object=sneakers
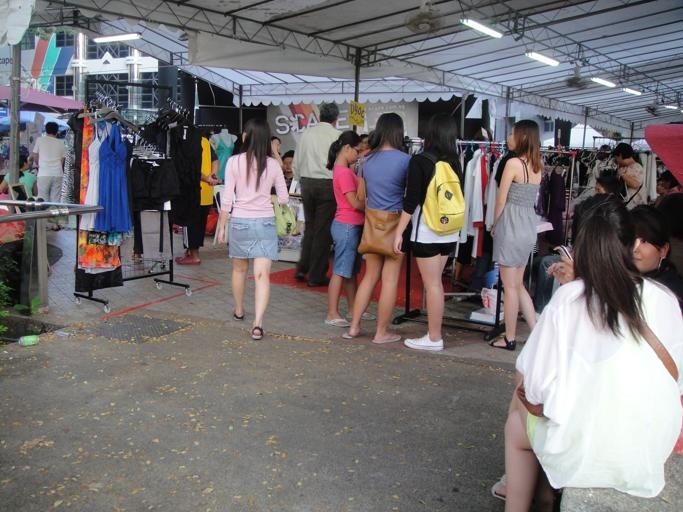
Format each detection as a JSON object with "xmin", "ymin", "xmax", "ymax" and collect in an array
[{"xmin": 402, "ymin": 331, "xmax": 445, "ymax": 352}]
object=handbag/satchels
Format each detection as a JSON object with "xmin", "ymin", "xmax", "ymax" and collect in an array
[
  {"xmin": 357, "ymin": 197, "xmax": 403, "ymax": 261},
  {"xmin": 270, "ymin": 194, "xmax": 297, "ymax": 239},
  {"xmin": 515, "ymin": 377, "xmax": 544, "ymax": 416}
]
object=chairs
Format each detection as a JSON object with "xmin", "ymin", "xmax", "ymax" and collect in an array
[{"xmin": 210, "ymin": 183, "xmax": 236, "ymax": 246}]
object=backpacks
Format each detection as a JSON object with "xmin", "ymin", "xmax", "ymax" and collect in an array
[{"xmin": 417, "ymin": 150, "xmax": 466, "ymax": 236}]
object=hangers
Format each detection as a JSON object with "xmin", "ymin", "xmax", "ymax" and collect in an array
[
  {"xmin": 452, "ymin": 138, "xmax": 600, "ymax": 167},
  {"xmin": 75, "ymin": 91, "xmax": 225, "ymax": 164}
]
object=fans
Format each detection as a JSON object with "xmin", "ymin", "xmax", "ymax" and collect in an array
[{"xmin": 403, "ymin": 0, "xmax": 445, "ymax": 36}]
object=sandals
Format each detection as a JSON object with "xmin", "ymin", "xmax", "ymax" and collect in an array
[
  {"xmin": 491, "ymin": 478, "xmax": 508, "ymax": 502},
  {"xmin": 250, "ymin": 326, "xmax": 265, "ymax": 339},
  {"xmin": 488, "ymin": 335, "xmax": 516, "ymax": 350},
  {"xmin": 233, "ymin": 308, "xmax": 246, "ymax": 321},
  {"xmin": 45, "ymin": 224, "xmax": 61, "ymax": 231}
]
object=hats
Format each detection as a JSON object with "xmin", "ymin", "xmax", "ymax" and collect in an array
[{"xmin": 626, "ymin": 204, "xmax": 671, "ymax": 249}]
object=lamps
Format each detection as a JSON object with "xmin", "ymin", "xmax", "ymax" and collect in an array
[
  {"xmin": 589, "ymin": 69, "xmax": 617, "ymax": 89},
  {"xmin": 621, "ymin": 80, "xmax": 641, "ymax": 97},
  {"xmin": 458, "ymin": 8, "xmax": 504, "ymax": 40},
  {"xmin": 91, "ymin": 20, "xmax": 148, "ymax": 44},
  {"xmin": 523, "ymin": 40, "xmax": 560, "ymax": 68}
]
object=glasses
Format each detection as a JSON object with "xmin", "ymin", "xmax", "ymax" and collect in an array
[{"xmin": 353, "ymin": 147, "xmax": 360, "ymax": 155}]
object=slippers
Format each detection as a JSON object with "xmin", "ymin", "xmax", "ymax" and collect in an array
[
  {"xmin": 346, "ymin": 312, "xmax": 376, "ymax": 320},
  {"xmin": 174, "ymin": 255, "xmax": 201, "ymax": 264},
  {"xmin": 342, "ymin": 328, "xmax": 368, "ymax": 339},
  {"xmin": 372, "ymin": 333, "xmax": 401, "ymax": 344},
  {"xmin": 324, "ymin": 316, "xmax": 351, "ymax": 327}
]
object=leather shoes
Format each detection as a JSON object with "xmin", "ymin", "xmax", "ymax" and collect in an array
[
  {"xmin": 307, "ymin": 277, "xmax": 330, "ymax": 287},
  {"xmin": 293, "ymin": 271, "xmax": 305, "ymax": 281}
]
